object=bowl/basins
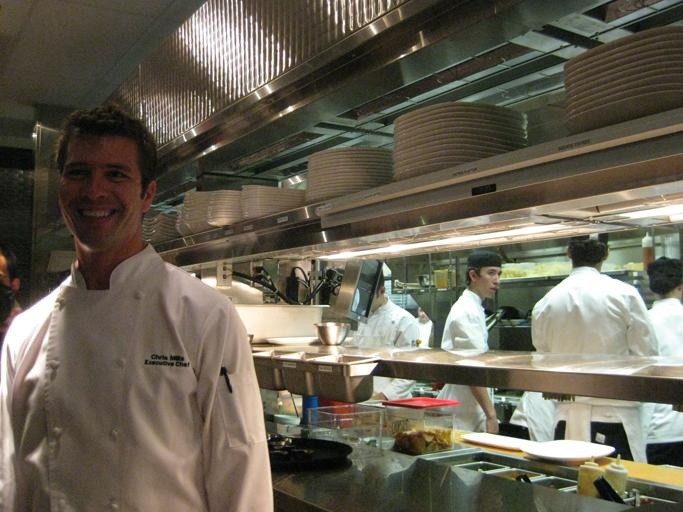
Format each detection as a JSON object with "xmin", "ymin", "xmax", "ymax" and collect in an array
[
  {"xmin": 363, "ymin": 437, "xmax": 395, "ymax": 450},
  {"xmin": 315, "ymin": 321, "xmax": 351, "ymax": 346}
]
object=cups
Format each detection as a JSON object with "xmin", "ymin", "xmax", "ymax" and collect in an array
[{"xmin": 272, "ymin": 413, "xmax": 300, "ymax": 426}]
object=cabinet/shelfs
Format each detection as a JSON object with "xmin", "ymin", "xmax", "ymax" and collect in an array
[
  {"xmin": 32, "ymin": 19, "xmax": 682, "ymax": 510},
  {"xmin": 398, "ymin": 224, "xmax": 682, "ymax": 467}
]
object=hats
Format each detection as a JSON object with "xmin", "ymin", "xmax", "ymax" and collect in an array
[{"xmin": 468, "ymin": 250, "xmax": 503, "ymax": 268}]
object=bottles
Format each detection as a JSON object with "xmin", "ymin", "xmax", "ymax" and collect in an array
[
  {"xmin": 641, "ymin": 231, "xmax": 652, "ymax": 271},
  {"xmin": 605, "ymin": 452, "xmax": 626, "ymax": 497},
  {"xmin": 577, "ymin": 455, "xmax": 602, "ymax": 499}
]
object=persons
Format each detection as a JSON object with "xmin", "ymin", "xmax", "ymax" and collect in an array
[
  {"xmin": 436, "ymin": 250, "xmax": 501, "ymax": 434},
  {"xmin": 350, "ymin": 268, "xmax": 419, "ymax": 403},
  {"xmin": 638, "ymin": 256, "xmax": 683, "ymax": 468},
  {"xmin": 416, "ymin": 307, "xmax": 433, "ymax": 351},
  {"xmin": 0, "ymin": 104, "xmax": 277, "ymax": 511},
  {"xmin": 524, "ymin": 233, "xmax": 659, "ymax": 464},
  {"xmin": 0, "ymin": 244, "xmax": 25, "ymax": 335}
]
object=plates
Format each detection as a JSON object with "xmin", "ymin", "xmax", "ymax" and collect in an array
[
  {"xmin": 389, "ymin": 101, "xmax": 527, "ymax": 180},
  {"xmin": 140, "ymin": 211, "xmax": 176, "ymax": 245},
  {"xmin": 520, "ymin": 438, "xmax": 615, "ymax": 464},
  {"xmin": 463, "ymin": 432, "xmax": 537, "ymax": 451},
  {"xmin": 265, "ymin": 337, "xmax": 318, "ymax": 346},
  {"xmin": 560, "ymin": 24, "xmax": 683, "ymax": 134},
  {"xmin": 302, "ymin": 145, "xmax": 389, "ymax": 205},
  {"xmin": 175, "ymin": 184, "xmax": 304, "ymax": 236}
]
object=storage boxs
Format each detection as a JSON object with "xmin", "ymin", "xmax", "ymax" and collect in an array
[
  {"xmin": 308, "ymin": 400, "xmax": 384, "ymax": 446},
  {"xmin": 383, "ymin": 396, "xmax": 459, "ymax": 455}
]
